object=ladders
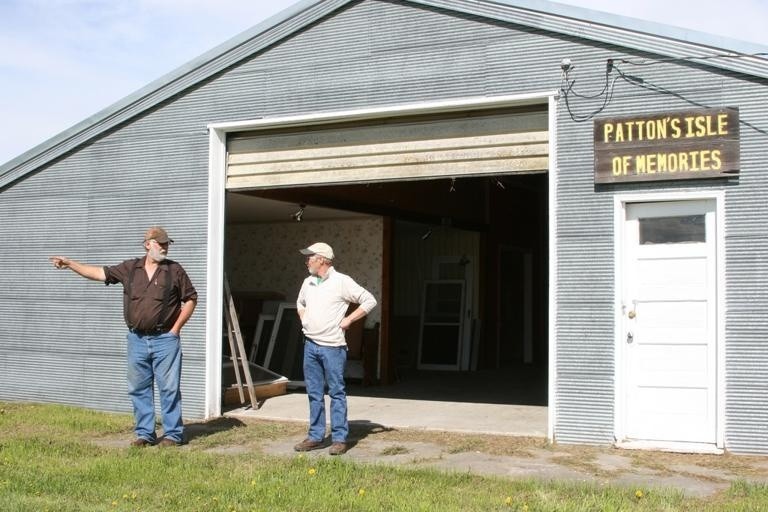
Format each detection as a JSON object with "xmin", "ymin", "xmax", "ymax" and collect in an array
[{"xmin": 224, "ymin": 273, "xmax": 258, "ymax": 410}]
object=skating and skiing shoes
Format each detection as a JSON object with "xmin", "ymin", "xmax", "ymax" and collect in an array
[{"xmin": 130, "ymin": 436, "xmax": 154, "ymax": 448}]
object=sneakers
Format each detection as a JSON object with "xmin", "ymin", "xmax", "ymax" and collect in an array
[
  {"xmin": 329, "ymin": 438, "xmax": 352, "ymax": 455},
  {"xmin": 293, "ymin": 435, "xmax": 327, "ymax": 451},
  {"xmin": 157, "ymin": 435, "xmax": 184, "ymax": 448}
]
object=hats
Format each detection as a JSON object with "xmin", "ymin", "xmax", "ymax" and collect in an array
[
  {"xmin": 296, "ymin": 241, "xmax": 338, "ymax": 261},
  {"xmin": 142, "ymin": 225, "xmax": 175, "ymax": 244}
]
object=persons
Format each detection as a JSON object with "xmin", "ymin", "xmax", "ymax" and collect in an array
[
  {"xmin": 48, "ymin": 225, "xmax": 198, "ymax": 447},
  {"xmin": 295, "ymin": 241, "xmax": 377, "ymax": 454}
]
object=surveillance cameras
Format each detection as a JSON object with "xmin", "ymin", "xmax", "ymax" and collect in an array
[{"xmin": 561, "ymin": 58, "xmax": 570, "ymax": 70}]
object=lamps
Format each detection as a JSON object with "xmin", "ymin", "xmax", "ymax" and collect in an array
[{"xmin": 288, "ymin": 201, "xmax": 306, "ymax": 222}]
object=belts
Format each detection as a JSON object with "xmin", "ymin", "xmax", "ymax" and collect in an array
[
  {"xmin": 303, "ymin": 334, "xmax": 313, "ymax": 342},
  {"xmin": 129, "ymin": 327, "xmax": 170, "ymax": 338}
]
